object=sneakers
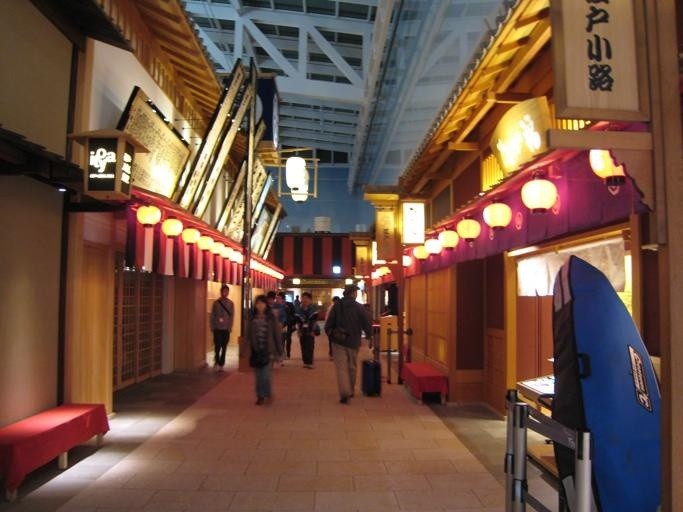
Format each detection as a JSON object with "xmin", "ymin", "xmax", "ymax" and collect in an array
[
  {"xmin": 304, "ymin": 364, "xmax": 313, "ymax": 369},
  {"xmin": 341, "ymin": 398, "xmax": 348, "ymax": 403},
  {"xmin": 255, "ymin": 396, "xmax": 264, "ymax": 406}
]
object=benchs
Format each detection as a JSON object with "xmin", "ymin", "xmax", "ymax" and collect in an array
[
  {"xmin": 0, "ymin": 402, "xmax": 110, "ymax": 501},
  {"xmin": 400, "ymin": 361, "xmax": 448, "ymax": 405}
]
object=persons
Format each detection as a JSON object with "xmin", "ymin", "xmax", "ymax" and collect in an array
[
  {"xmin": 265, "ymin": 291, "xmax": 286, "ymax": 367},
  {"xmin": 323, "ymin": 286, "xmax": 374, "ymax": 402},
  {"xmin": 211, "ymin": 286, "xmax": 234, "ymax": 373},
  {"xmin": 277, "ymin": 293, "xmax": 294, "ymax": 359},
  {"xmin": 295, "ymin": 296, "xmax": 300, "ymax": 312},
  {"xmin": 295, "ymin": 292, "xmax": 319, "ymax": 368},
  {"xmin": 243, "ymin": 294, "xmax": 281, "ymax": 405},
  {"xmin": 326, "ymin": 292, "xmax": 340, "ymax": 361}
]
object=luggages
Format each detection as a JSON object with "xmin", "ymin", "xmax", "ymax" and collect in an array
[{"xmin": 361, "ymin": 360, "xmax": 381, "ymax": 397}]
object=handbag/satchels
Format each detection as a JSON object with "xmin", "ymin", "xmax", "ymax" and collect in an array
[
  {"xmin": 314, "ymin": 325, "xmax": 320, "ymax": 336},
  {"xmin": 250, "ymin": 350, "xmax": 268, "ymax": 368},
  {"xmin": 332, "ymin": 328, "xmax": 348, "ymax": 346}
]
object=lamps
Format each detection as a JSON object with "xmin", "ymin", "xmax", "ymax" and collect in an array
[
  {"xmin": 372, "ymin": 149, "xmax": 626, "ymax": 277},
  {"xmin": 67, "ymin": 128, "xmax": 151, "ymax": 201},
  {"xmin": 136, "ymin": 205, "xmax": 284, "ymax": 280}
]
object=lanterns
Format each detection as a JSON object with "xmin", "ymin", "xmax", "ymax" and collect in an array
[
  {"xmin": 181, "ymin": 227, "xmax": 200, "ymax": 247},
  {"xmin": 213, "ymin": 242, "xmax": 289, "ymax": 281},
  {"xmin": 161, "ymin": 217, "xmax": 185, "ymax": 239},
  {"xmin": 286, "ymin": 156, "xmax": 309, "ymax": 204},
  {"xmin": 520, "ymin": 180, "xmax": 557, "ymax": 214},
  {"xmin": 482, "ymin": 204, "xmax": 512, "ymax": 231},
  {"xmin": 438, "ymin": 230, "xmax": 457, "ymax": 251},
  {"xmin": 589, "ymin": 148, "xmax": 625, "ymax": 189},
  {"xmin": 136, "ymin": 202, "xmax": 162, "ymax": 227},
  {"xmin": 198, "ymin": 234, "xmax": 215, "ymax": 251},
  {"xmin": 367, "ymin": 237, "xmax": 442, "ymax": 280},
  {"xmin": 455, "ymin": 219, "xmax": 481, "ymax": 242}
]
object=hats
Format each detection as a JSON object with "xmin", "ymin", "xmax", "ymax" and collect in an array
[{"xmin": 344, "ymin": 285, "xmax": 361, "ymax": 293}]
